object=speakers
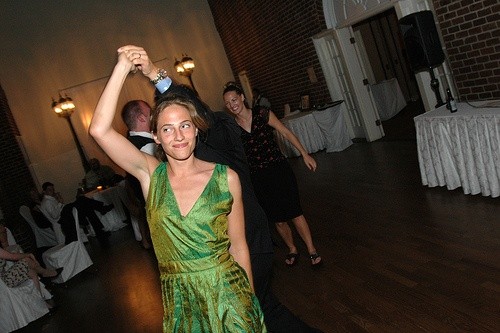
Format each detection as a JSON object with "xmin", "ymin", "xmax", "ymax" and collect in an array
[{"xmin": 398, "ymin": 10, "xmax": 445, "ymax": 74}]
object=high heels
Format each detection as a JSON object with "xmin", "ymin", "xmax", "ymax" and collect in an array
[{"xmin": 44, "ymin": 267, "xmax": 64, "ymax": 282}]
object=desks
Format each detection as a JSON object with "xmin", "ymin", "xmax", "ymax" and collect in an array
[
  {"xmin": 272, "ymin": 110, "xmax": 326, "ymax": 157},
  {"xmin": 413, "ymin": 100, "xmax": 499, "ymax": 199},
  {"xmin": 85, "ymin": 184, "xmax": 128, "ymax": 231}
]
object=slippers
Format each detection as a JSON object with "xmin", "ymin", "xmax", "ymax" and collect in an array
[
  {"xmin": 285, "ymin": 252, "xmax": 300, "ymax": 267},
  {"xmin": 310, "ymin": 252, "xmax": 322, "ymax": 270}
]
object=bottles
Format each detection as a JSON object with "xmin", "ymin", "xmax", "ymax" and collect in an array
[{"xmin": 446, "ymin": 89, "xmax": 457, "ymax": 113}]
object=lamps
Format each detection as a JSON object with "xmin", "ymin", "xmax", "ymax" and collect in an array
[
  {"xmin": 174, "ymin": 52, "xmax": 195, "ymax": 77},
  {"xmin": 51, "ymin": 93, "xmax": 76, "ymax": 117}
]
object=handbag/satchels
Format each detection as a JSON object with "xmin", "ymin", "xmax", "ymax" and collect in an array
[{"xmin": 3, "ymin": 261, "xmax": 30, "ymax": 287}]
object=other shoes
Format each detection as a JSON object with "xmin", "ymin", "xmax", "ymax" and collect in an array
[
  {"xmin": 100, "ymin": 203, "xmax": 115, "ymax": 215},
  {"xmin": 96, "ymin": 230, "xmax": 111, "ymax": 238},
  {"xmin": 141, "ymin": 241, "xmax": 152, "ymax": 251}
]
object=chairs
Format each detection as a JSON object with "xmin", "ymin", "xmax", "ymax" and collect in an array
[
  {"xmin": 36, "ymin": 203, "xmax": 94, "ymax": 243},
  {"xmin": 42, "ymin": 208, "xmax": 94, "ymax": 283},
  {"xmin": 117, "ymin": 179, "xmax": 143, "ymax": 241},
  {"xmin": 18, "ymin": 205, "xmax": 55, "ymax": 246},
  {"xmin": 0, "ymin": 227, "xmax": 53, "ymax": 333}
]
